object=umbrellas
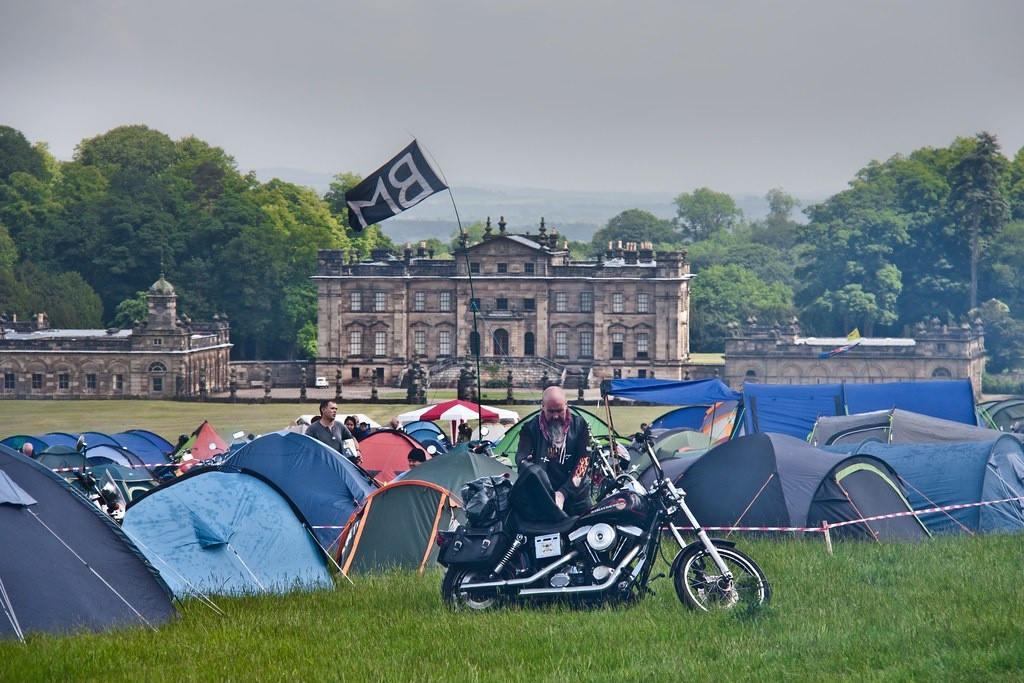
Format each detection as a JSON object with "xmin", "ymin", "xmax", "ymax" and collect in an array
[{"xmin": 398, "ymin": 399, "xmax": 519, "ymax": 445}]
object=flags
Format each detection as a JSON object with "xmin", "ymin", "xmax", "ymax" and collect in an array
[{"xmin": 344, "ymin": 140, "xmax": 448, "ymax": 234}]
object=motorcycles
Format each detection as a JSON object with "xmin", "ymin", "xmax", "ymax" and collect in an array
[{"xmin": 435, "ymin": 422, "xmax": 773, "ymax": 624}]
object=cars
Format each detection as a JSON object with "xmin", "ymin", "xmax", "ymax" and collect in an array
[{"xmin": 315, "ymin": 376, "xmax": 330, "ymax": 389}]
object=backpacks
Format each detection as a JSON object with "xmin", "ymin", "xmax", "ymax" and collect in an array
[{"xmin": 461, "ymin": 471, "xmax": 513, "ymax": 528}]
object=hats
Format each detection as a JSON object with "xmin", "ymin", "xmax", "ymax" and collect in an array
[{"xmin": 407, "ymin": 448, "xmax": 426, "ymax": 461}]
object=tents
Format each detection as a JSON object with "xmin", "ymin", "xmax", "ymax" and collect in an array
[
  {"xmin": 493, "ymin": 404, "xmax": 619, "ymax": 473},
  {"xmin": 600, "ymin": 377, "xmax": 1024, "ymax": 544},
  {"xmin": 0, "ymin": 442, "xmax": 179, "ymax": 643},
  {"xmin": 337, "ymin": 451, "xmax": 520, "ymax": 572},
  {"xmin": 285, "ymin": 413, "xmax": 381, "ymax": 434},
  {"xmin": 403, "ymin": 421, "xmax": 453, "ymax": 456},
  {"xmin": 355, "ymin": 429, "xmax": 434, "ymax": 485},
  {"xmin": 0, "ymin": 421, "xmax": 378, "ymax": 596}
]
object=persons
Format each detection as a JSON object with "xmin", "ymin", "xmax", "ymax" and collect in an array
[
  {"xmin": 170, "ymin": 434, "xmax": 189, "ymax": 456},
  {"xmin": 641, "ymin": 423, "xmax": 652, "ymax": 435},
  {"xmin": 22, "ymin": 442, "xmax": 34, "ymax": 457},
  {"xmin": 407, "ymin": 449, "xmax": 427, "ymax": 469},
  {"xmin": 516, "ymin": 386, "xmax": 594, "ymax": 518},
  {"xmin": 343, "ymin": 415, "xmax": 370, "ymax": 442},
  {"xmin": 390, "ymin": 418, "xmax": 408, "ymax": 434},
  {"xmin": 305, "ymin": 400, "xmax": 363, "ymax": 466}
]
